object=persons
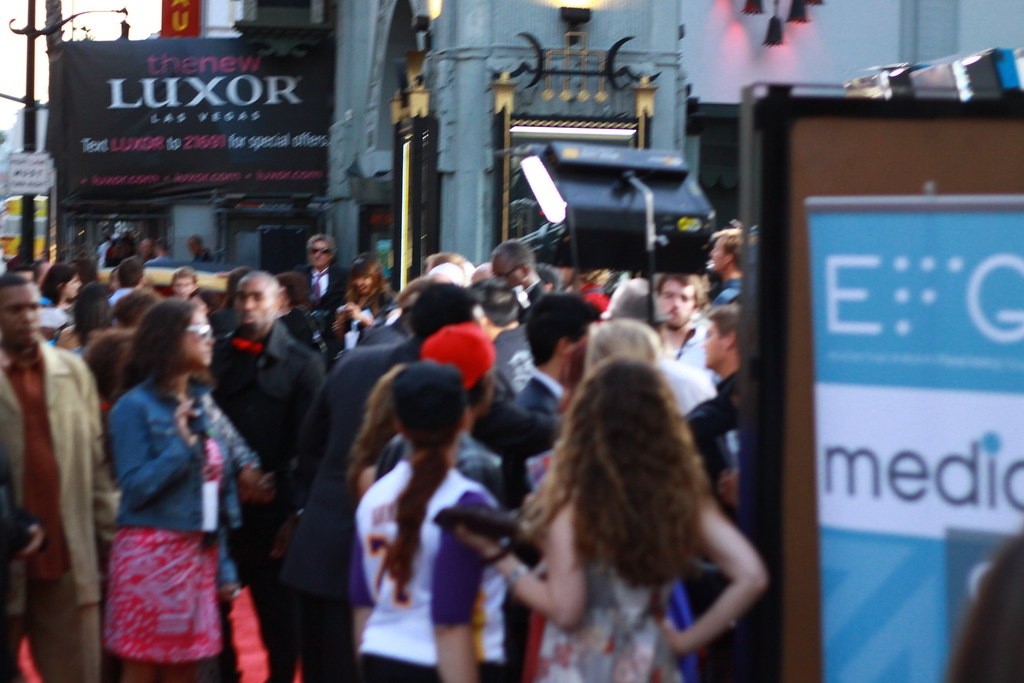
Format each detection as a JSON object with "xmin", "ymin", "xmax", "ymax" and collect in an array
[
  {"xmin": 103, "ymin": 297, "xmax": 277, "ymax": 683},
  {"xmin": 451, "ymin": 354, "xmax": 771, "ymax": 683},
  {"xmin": 0, "ymin": 222, "xmax": 770, "ymax": 683}
]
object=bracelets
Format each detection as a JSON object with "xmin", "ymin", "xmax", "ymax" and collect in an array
[{"xmin": 483, "ymin": 544, "xmax": 510, "ymax": 564}]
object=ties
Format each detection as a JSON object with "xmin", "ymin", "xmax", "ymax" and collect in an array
[{"xmin": 314, "ymin": 270, "xmax": 329, "ymax": 298}]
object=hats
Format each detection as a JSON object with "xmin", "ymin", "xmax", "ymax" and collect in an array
[
  {"xmin": 393, "ymin": 360, "xmax": 474, "ymax": 429},
  {"xmin": 421, "ymin": 323, "xmax": 497, "ymax": 391}
]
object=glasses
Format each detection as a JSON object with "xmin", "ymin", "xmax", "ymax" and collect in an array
[
  {"xmin": 188, "ymin": 324, "xmax": 213, "ymax": 339},
  {"xmin": 311, "ymin": 247, "xmax": 330, "ymax": 253},
  {"xmin": 495, "ymin": 265, "xmax": 520, "ymax": 280}
]
